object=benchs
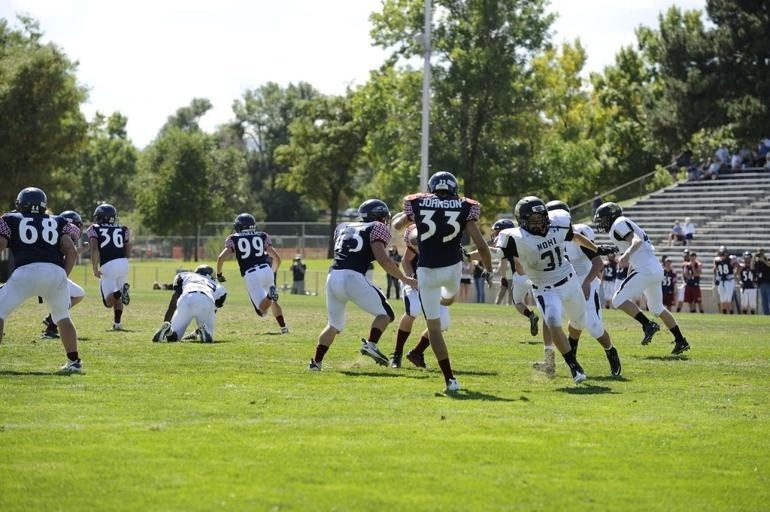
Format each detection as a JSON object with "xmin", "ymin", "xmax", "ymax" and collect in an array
[{"xmin": 591, "ymin": 169, "xmax": 770, "ymax": 288}]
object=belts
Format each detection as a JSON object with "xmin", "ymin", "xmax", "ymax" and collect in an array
[
  {"xmin": 532, "ymin": 273, "xmax": 572, "ymax": 289},
  {"xmin": 248, "ymin": 265, "xmax": 267, "ymax": 273}
]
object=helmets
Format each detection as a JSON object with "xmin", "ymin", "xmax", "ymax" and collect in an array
[
  {"xmin": 196, "ymin": 266, "xmax": 216, "ymax": 280},
  {"xmin": 234, "ymin": 213, "xmax": 256, "ymax": 234},
  {"xmin": 16, "ymin": 187, "xmax": 116, "ymax": 229},
  {"xmin": 357, "ymin": 172, "xmax": 623, "ymax": 237},
  {"xmin": 663, "ymin": 245, "xmax": 765, "ymax": 263}
]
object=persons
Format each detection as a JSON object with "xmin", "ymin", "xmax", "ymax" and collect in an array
[
  {"xmin": 665, "ymin": 134, "xmax": 770, "ymax": 184},
  {"xmin": 385, "ymin": 211, "xmax": 769, "ymax": 379},
  {"xmin": 289, "ymin": 254, "xmax": 306, "ymax": 296},
  {"xmin": 306, "ymin": 197, "xmax": 418, "ymax": 371},
  {"xmin": 0, "ymin": 186, "xmax": 174, "ymax": 377},
  {"xmin": 541, "ymin": 200, "xmax": 622, "ymax": 378},
  {"xmin": 215, "ymin": 212, "xmax": 289, "ymax": 334},
  {"xmin": 390, "ymin": 171, "xmax": 493, "ymax": 392},
  {"xmin": 591, "ymin": 201, "xmax": 691, "ymax": 356},
  {"xmin": 152, "ymin": 265, "xmax": 228, "ymax": 343},
  {"xmin": 495, "ymin": 194, "xmax": 614, "ymax": 384},
  {"xmin": 593, "ymin": 190, "xmax": 603, "ymax": 209}
]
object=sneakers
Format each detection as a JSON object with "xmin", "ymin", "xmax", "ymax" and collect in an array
[{"xmin": 39, "ymin": 284, "xmax": 291, "ymax": 374}]
